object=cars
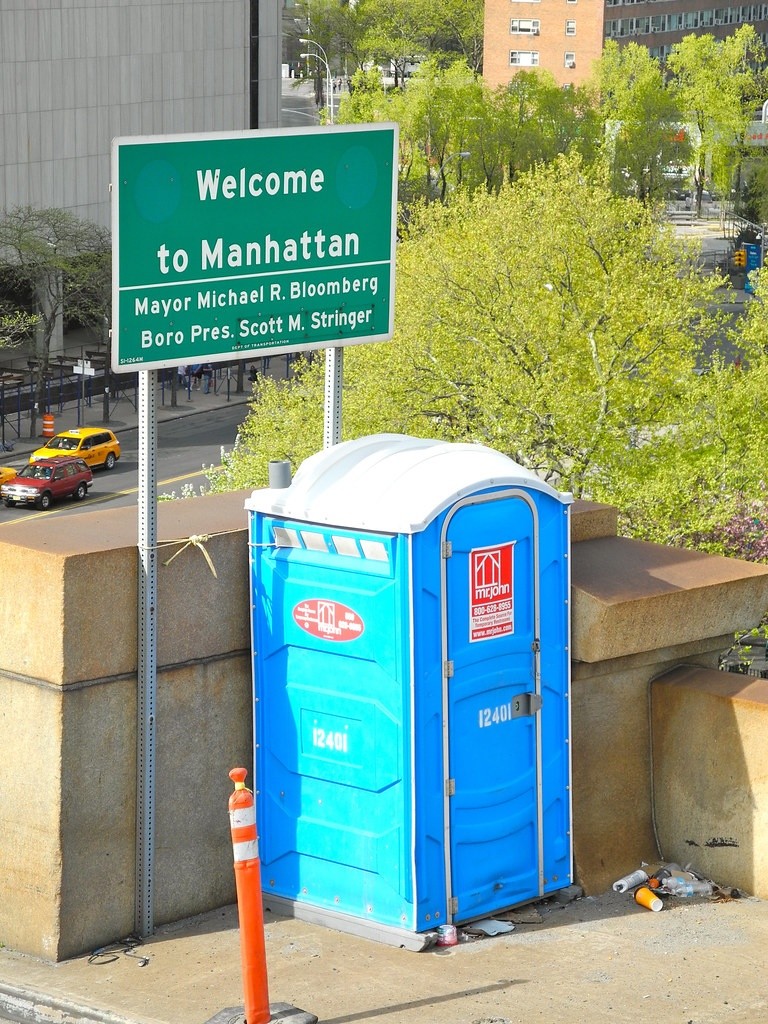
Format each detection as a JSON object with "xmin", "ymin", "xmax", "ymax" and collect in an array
[{"xmin": 0, "ymin": 466, "xmax": 19, "ymax": 498}]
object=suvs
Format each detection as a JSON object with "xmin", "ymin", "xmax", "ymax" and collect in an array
[
  {"xmin": 28, "ymin": 425, "xmax": 121, "ymax": 471},
  {"xmin": 0, "ymin": 455, "xmax": 93, "ymax": 511}
]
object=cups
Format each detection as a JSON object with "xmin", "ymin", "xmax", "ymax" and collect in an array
[
  {"xmin": 437, "ymin": 925, "xmax": 457, "ymax": 947},
  {"xmin": 634, "ymin": 885, "xmax": 663, "ymax": 912}
]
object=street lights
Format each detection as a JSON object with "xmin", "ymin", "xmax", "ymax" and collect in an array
[
  {"xmin": 755, "ymin": 222, "xmax": 766, "ymax": 276},
  {"xmin": 299, "ymin": 38, "xmax": 329, "ymax": 127},
  {"xmin": 300, "ymin": 52, "xmax": 334, "ymax": 126}
]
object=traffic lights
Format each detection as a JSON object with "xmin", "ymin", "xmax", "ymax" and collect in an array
[{"xmin": 734, "ymin": 249, "xmax": 747, "ymax": 266}]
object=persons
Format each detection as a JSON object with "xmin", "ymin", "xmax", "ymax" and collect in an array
[
  {"xmin": 177, "ymin": 363, "xmax": 212, "ymax": 394},
  {"xmin": 247, "ymin": 365, "xmax": 259, "ymax": 383}
]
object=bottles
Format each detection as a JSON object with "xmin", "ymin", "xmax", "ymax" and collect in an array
[
  {"xmin": 648, "ymin": 862, "xmax": 681, "ymax": 889},
  {"xmin": 671, "ymin": 884, "xmax": 713, "ymax": 898},
  {"xmin": 662, "ymin": 877, "xmax": 707, "ymax": 889},
  {"xmin": 613, "ymin": 870, "xmax": 648, "ymax": 893}
]
